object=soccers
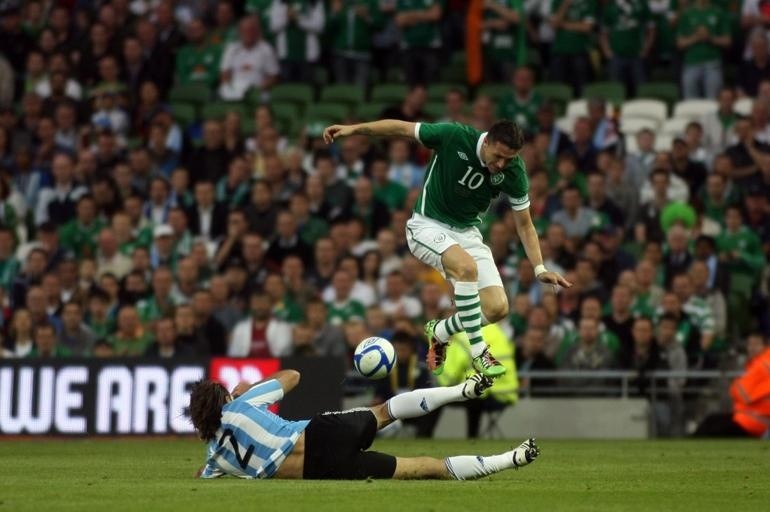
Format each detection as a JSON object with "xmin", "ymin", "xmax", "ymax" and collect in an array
[{"xmin": 354, "ymin": 337, "xmax": 397, "ymax": 379}]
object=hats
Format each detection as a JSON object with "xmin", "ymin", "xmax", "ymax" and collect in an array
[{"xmin": 153, "ymin": 225, "xmax": 174, "ymax": 237}]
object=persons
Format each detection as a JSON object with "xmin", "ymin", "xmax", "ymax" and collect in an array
[
  {"xmin": 0, "ymin": 1, "xmax": 769, "ymax": 438},
  {"xmin": 186, "ymin": 370, "xmax": 540, "ymax": 480}
]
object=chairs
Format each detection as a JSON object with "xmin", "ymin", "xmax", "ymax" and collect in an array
[{"xmin": 162, "ymin": 41, "xmax": 740, "ymax": 174}]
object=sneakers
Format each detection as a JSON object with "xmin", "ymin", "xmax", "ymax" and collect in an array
[
  {"xmin": 462, "ymin": 345, "xmax": 506, "ymax": 399},
  {"xmin": 424, "ymin": 319, "xmax": 447, "ymax": 375},
  {"xmin": 513, "ymin": 438, "xmax": 540, "ymax": 466}
]
